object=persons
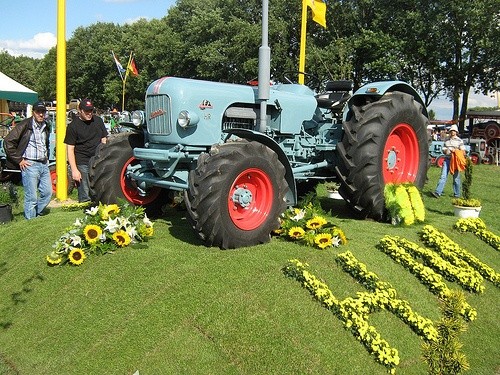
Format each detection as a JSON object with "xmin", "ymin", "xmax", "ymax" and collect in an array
[
  {"xmin": 110, "ymin": 108, "xmax": 119, "ymax": 134},
  {"xmin": 64, "ymin": 99, "xmax": 108, "ymax": 203},
  {"xmin": 3, "ymin": 102, "xmax": 52, "ymax": 219},
  {"xmin": 430, "ymin": 123, "xmax": 465, "ymax": 198},
  {"xmin": 431, "ymin": 129, "xmax": 449, "ymax": 141}
]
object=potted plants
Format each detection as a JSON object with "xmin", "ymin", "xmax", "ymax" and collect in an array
[{"xmin": 327, "ymin": 185, "xmax": 343, "ymax": 200}]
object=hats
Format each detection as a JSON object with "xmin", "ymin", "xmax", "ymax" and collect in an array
[
  {"xmin": 449, "ymin": 124, "xmax": 458, "ymax": 132},
  {"xmin": 33, "ymin": 102, "xmax": 46, "ymax": 111},
  {"xmin": 79, "ymin": 99, "xmax": 94, "ymax": 110}
]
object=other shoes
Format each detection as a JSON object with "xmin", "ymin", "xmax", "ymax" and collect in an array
[
  {"xmin": 430, "ymin": 192, "xmax": 440, "ymax": 199},
  {"xmin": 454, "ymin": 196, "xmax": 459, "ymax": 198}
]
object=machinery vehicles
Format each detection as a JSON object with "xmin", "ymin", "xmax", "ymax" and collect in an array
[
  {"xmin": 0, "ymin": 112, "xmax": 77, "ymax": 196},
  {"xmin": 429, "ymin": 124, "xmax": 495, "ymax": 169},
  {"xmin": 87, "ymin": 1, "xmax": 430, "ymax": 251}
]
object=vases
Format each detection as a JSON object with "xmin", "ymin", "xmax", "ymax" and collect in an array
[
  {"xmin": 0, "ymin": 204, "xmax": 13, "ymax": 223},
  {"xmin": 455, "ymin": 205, "xmax": 481, "ymax": 218}
]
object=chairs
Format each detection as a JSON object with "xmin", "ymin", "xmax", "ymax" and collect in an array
[{"xmin": 317, "ymin": 80, "xmax": 353, "ymax": 109}]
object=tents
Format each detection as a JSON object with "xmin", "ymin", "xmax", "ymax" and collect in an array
[{"xmin": 0, "ymin": 72, "xmax": 38, "ymax": 105}]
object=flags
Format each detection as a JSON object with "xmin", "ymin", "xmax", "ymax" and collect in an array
[
  {"xmin": 115, "ymin": 59, "xmax": 126, "ymax": 72},
  {"xmin": 302, "ymin": 0, "xmax": 327, "ymax": 30},
  {"xmin": 127, "ymin": 59, "xmax": 138, "ymax": 76}
]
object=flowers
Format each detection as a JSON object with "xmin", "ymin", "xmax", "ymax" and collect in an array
[
  {"xmin": 0, "ymin": 181, "xmax": 20, "ymax": 208},
  {"xmin": 451, "ymin": 197, "xmax": 482, "ymax": 207}
]
